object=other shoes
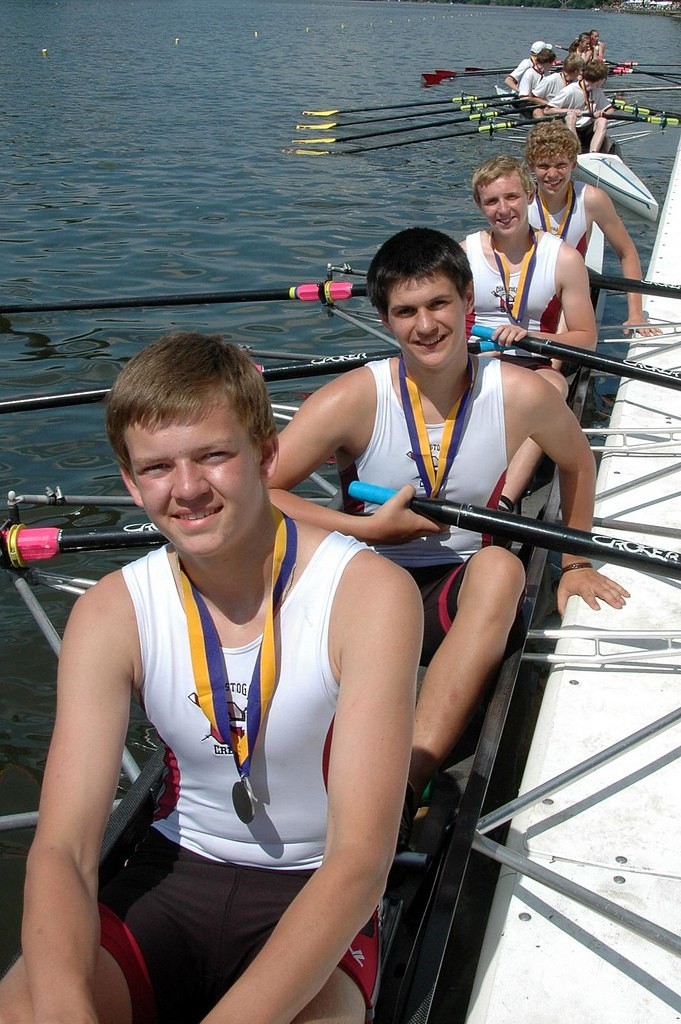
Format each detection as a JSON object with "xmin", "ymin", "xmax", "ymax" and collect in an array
[
  {"xmin": 394, "ymin": 781, "xmax": 422, "ymax": 855},
  {"xmin": 492, "ymin": 495, "xmax": 515, "ymax": 550}
]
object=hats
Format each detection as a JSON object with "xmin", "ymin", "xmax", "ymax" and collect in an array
[{"xmin": 530, "ymin": 41, "xmax": 552, "ymax": 56}]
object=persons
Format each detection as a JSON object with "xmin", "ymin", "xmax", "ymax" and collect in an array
[
  {"xmin": 569, "ymin": 31, "xmax": 604, "ymax": 66},
  {"xmin": 505, "ymin": 41, "xmax": 553, "ymax": 108},
  {"xmin": 544, "ymin": 59, "xmax": 615, "ymax": 154},
  {"xmin": 268, "ymin": 228, "xmax": 630, "ymax": 851},
  {"xmin": 0, "ymin": 333, "xmax": 425, "ymax": 1024},
  {"xmin": 620, "ymin": 2, "xmax": 681, "ymax": 12},
  {"xmin": 524, "ymin": 121, "xmax": 663, "ymax": 369},
  {"xmin": 456, "ymin": 156, "xmax": 597, "ymax": 547},
  {"xmin": 530, "ymin": 53, "xmax": 584, "ymax": 119},
  {"xmin": 519, "ymin": 49, "xmax": 555, "ymax": 119}
]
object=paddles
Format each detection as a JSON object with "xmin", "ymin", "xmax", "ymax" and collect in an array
[
  {"xmin": 295, "ymin": 98, "xmax": 524, "ymax": 130},
  {"xmin": 348, "ymin": 481, "xmax": 681, "ymax": 581},
  {"xmin": 289, "ymin": 103, "xmax": 546, "ymax": 146},
  {"xmin": 0, "ymin": 261, "xmax": 371, "ymax": 317},
  {"xmin": 571, "ymin": 97, "xmax": 681, "ymax": 128},
  {"xmin": 419, "ymin": 66, "xmax": 518, "ymax": 90},
  {"xmin": 585, "ymin": 271, "xmax": 681, "ymax": 299},
  {"xmin": 554, "ymin": 43, "xmax": 681, "ymax": 85},
  {"xmin": 0, "ymin": 335, "xmax": 515, "ymax": 415},
  {"xmin": 4, "ymin": 517, "xmax": 176, "ymax": 569},
  {"xmin": 301, "ymin": 93, "xmax": 515, "ymax": 117},
  {"xmin": 471, "ymin": 324, "xmax": 681, "ymax": 395},
  {"xmin": 281, "ymin": 112, "xmax": 566, "ymax": 158}
]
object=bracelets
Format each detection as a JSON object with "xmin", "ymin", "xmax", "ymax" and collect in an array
[{"xmin": 561, "ymin": 563, "xmax": 592, "ymax": 573}]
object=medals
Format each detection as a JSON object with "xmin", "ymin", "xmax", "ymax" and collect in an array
[{"xmin": 232, "ymin": 777, "xmax": 256, "ymax": 824}]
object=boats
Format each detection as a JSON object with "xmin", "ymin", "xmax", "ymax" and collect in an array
[
  {"xmin": 100, "ymin": 222, "xmax": 604, "ymax": 1023},
  {"xmin": 495, "ymin": 89, "xmax": 659, "ymax": 221}
]
